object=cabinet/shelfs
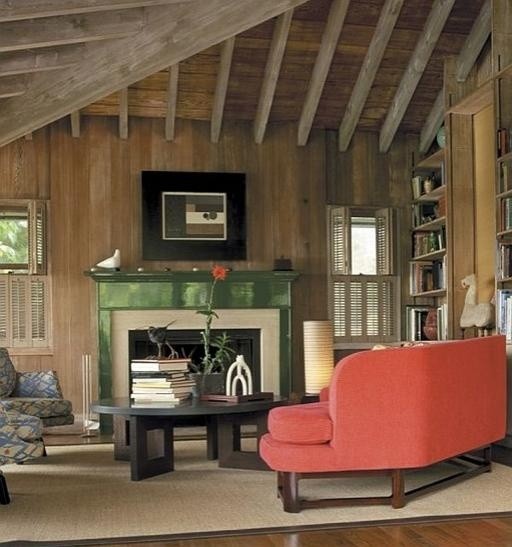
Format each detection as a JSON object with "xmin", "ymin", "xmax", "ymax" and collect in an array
[
  {"xmin": 403, "ymin": 143, "xmax": 454, "ymax": 341},
  {"xmin": 490, "ymin": 79, "xmax": 511, "ymax": 468}
]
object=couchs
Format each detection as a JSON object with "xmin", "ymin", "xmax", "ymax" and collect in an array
[
  {"xmin": 259, "ymin": 334, "xmax": 506, "ymax": 511},
  {"xmin": 0, "ymin": 348, "xmax": 75, "ymax": 508}
]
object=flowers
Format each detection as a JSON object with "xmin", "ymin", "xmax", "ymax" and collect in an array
[{"xmin": 183, "ymin": 262, "xmax": 238, "ymax": 373}]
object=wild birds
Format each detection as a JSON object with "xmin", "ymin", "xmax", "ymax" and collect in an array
[{"xmin": 147, "ymin": 319, "xmax": 180, "ymax": 361}]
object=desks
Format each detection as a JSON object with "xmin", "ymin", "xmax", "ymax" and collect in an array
[{"xmin": 88, "ymin": 393, "xmax": 278, "ymax": 483}]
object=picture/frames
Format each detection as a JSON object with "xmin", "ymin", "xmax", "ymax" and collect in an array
[{"xmin": 162, "ymin": 191, "xmax": 228, "ymax": 242}]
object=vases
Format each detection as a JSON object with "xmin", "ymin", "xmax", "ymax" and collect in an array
[{"xmin": 198, "ymin": 372, "xmax": 232, "ymax": 397}]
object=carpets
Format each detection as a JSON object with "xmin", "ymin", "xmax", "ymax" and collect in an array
[{"xmin": 2, "ymin": 436, "xmax": 510, "ymax": 546}]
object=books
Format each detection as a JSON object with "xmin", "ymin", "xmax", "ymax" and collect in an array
[
  {"xmin": 406, "ymin": 125, "xmax": 512, "ymax": 350},
  {"xmin": 129, "ymin": 353, "xmax": 197, "ymax": 408}
]
